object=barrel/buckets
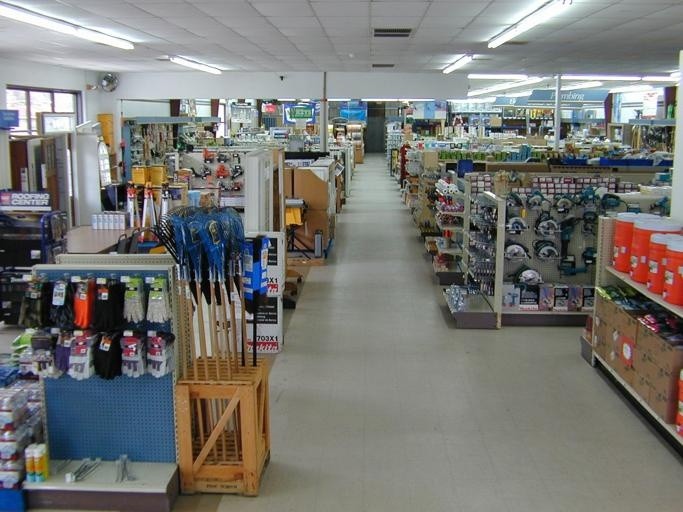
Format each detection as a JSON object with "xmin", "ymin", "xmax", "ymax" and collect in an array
[
  {"xmin": 646, "ymin": 234, "xmax": 681, "ymax": 295},
  {"xmin": 612, "ymin": 212, "xmax": 662, "ymax": 273},
  {"xmin": 628, "ymin": 219, "xmax": 683, "ymax": 282},
  {"xmin": 662, "ymin": 242, "xmax": 683, "ymax": 305},
  {"xmin": 674, "ymin": 369, "xmax": 682, "ymax": 437}
]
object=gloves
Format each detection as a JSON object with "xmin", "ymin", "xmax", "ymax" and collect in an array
[{"xmin": 1, "ymin": 276, "xmax": 174, "ymax": 383}]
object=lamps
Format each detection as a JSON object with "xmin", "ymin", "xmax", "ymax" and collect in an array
[
  {"xmin": 441, "ymin": 0, "xmax": 572, "ymax": 75},
  {"xmin": 0, "ymin": 0, "xmax": 221, "ymax": 76}
]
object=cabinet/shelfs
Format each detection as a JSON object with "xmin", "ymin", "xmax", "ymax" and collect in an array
[
  {"xmin": 0, "ymin": 116, "xmax": 366, "ymax": 269},
  {"xmin": 1, "ymin": 139, "xmax": 289, "ymax": 512},
  {"xmin": 386, "ymin": 121, "xmax": 683, "ymax": 333},
  {"xmin": 581, "ymin": 209, "xmax": 683, "ymax": 457}
]
